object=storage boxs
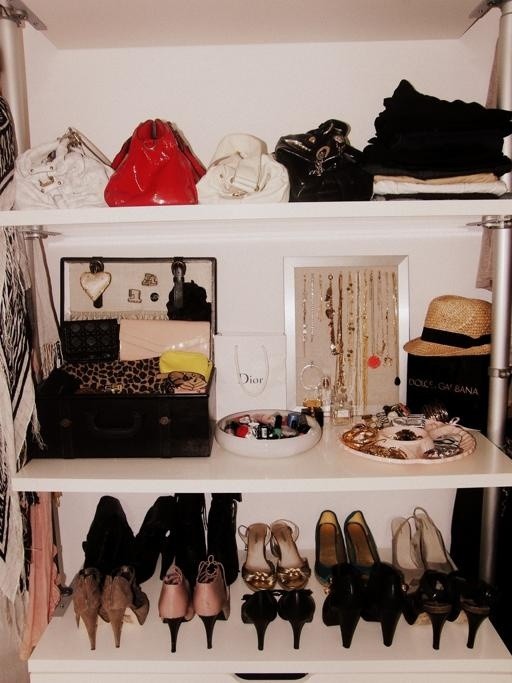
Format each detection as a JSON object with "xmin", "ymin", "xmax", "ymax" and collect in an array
[{"xmin": 406, "ymin": 343, "xmax": 491, "ymax": 433}]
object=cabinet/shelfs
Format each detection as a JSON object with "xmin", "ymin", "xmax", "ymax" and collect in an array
[{"xmin": 1, "ymin": 0, "xmax": 512, "ymax": 682}]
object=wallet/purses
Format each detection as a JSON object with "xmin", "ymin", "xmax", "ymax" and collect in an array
[
  {"xmin": 118, "ymin": 319, "xmax": 210, "ymax": 361},
  {"xmin": 60, "ymin": 318, "xmax": 120, "ymax": 364},
  {"xmin": 159, "ymin": 350, "xmax": 213, "ymax": 383},
  {"xmin": 60, "ymin": 356, "xmax": 163, "ymax": 395}
]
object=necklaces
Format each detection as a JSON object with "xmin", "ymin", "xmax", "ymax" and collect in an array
[{"xmin": 301, "ymin": 270, "xmax": 401, "ymax": 415}]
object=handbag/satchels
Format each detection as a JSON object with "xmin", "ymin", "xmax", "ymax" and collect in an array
[
  {"xmin": 196, "ymin": 133, "xmax": 291, "ymax": 205},
  {"xmin": 213, "ymin": 331, "xmax": 287, "ymax": 419},
  {"xmin": 276, "ymin": 118, "xmax": 374, "ymax": 202},
  {"xmin": 361, "ymin": 79, "xmax": 512, "ymax": 181},
  {"xmin": 104, "ymin": 118, "xmax": 206, "ymax": 207},
  {"xmin": 15, "ymin": 127, "xmax": 116, "ymax": 209}
]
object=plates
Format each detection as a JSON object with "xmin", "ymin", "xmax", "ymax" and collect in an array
[{"xmin": 336, "ymin": 415, "xmax": 478, "ymax": 466}]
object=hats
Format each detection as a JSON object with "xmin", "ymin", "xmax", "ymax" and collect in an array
[{"xmin": 403, "ymin": 295, "xmax": 491, "ymax": 357}]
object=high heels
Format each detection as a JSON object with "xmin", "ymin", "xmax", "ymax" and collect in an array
[
  {"xmin": 73, "ymin": 492, "xmax": 243, "ymax": 653},
  {"xmin": 238, "ymin": 506, "xmax": 491, "ymax": 650}
]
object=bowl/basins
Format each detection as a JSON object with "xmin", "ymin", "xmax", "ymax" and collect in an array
[{"xmin": 215, "ymin": 409, "xmax": 321, "ymax": 459}]
